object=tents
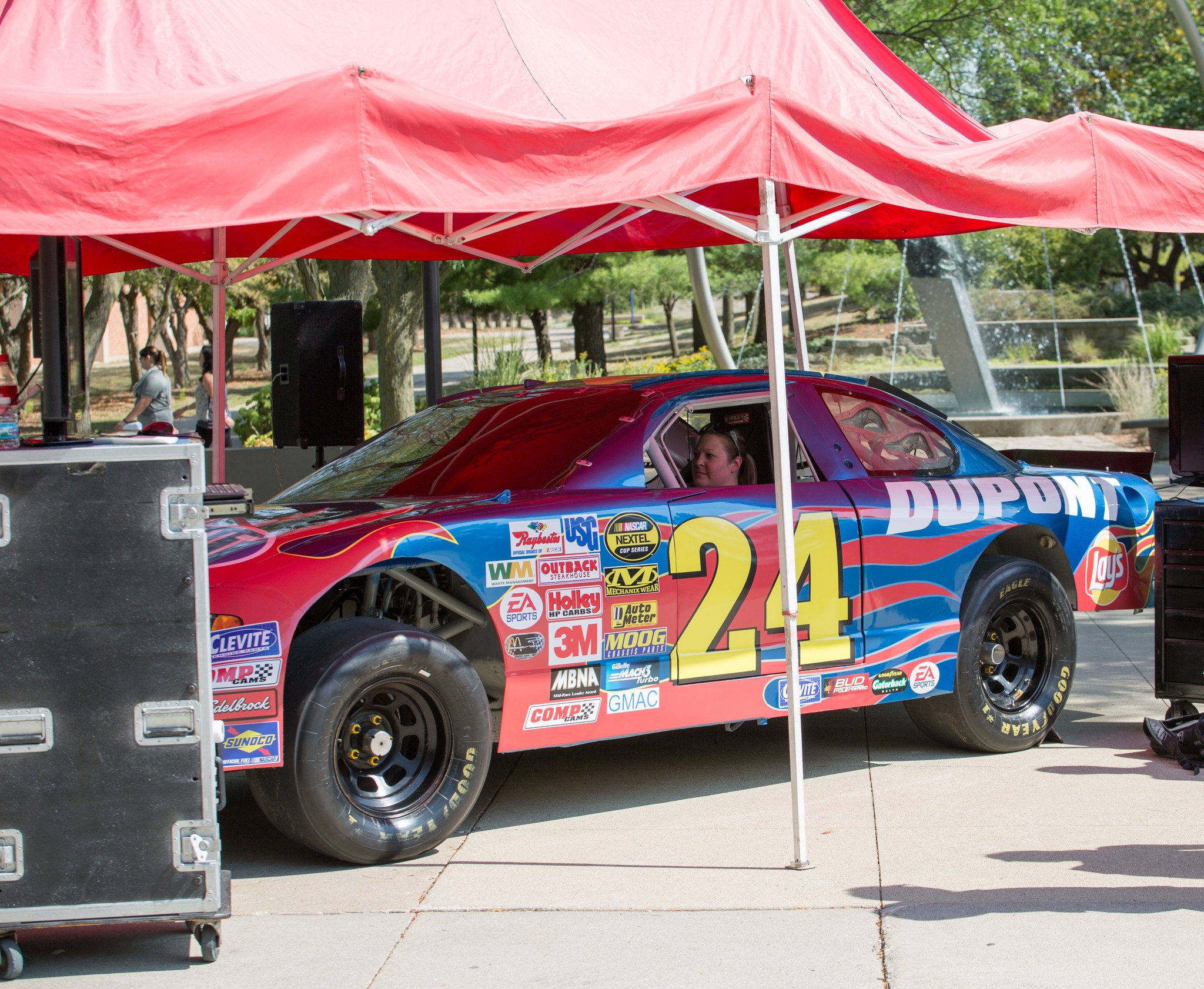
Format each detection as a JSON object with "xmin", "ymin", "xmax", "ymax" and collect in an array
[{"xmin": 0, "ymin": 1, "xmax": 1204, "ymax": 871}]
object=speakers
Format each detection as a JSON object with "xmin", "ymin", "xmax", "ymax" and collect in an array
[
  {"xmin": 271, "ymin": 300, "xmax": 365, "ymax": 446},
  {"xmin": 1169, "ymin": 353, "xmax": 1204, "ymax": 478},
  {"xmin": 1153, "ymin": 497, "xmax": 1204, "ymax": 702}
]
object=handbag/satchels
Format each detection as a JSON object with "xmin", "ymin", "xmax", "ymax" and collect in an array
[{"xmin": 196, "ymin": 421, "xmax": 232, "ymax": 448}]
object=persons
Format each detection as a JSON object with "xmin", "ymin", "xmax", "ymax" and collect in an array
[
  {"xmin": 171, "ymin": 345, "xmax": 235, "ymax": 448},
  {"xmin": 685, "ymin": 419, "xmax": 759, "ymax": 486},
  {"xmin": 112, "ymin": 346, "xmax": 174, "ymax": 433}
]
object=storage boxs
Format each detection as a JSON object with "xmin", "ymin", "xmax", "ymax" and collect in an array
[{"xmin": 0, "ymin": 444, "xmax": 221, "ymax": 923}]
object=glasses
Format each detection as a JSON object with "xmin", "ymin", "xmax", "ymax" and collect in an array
[
  {"xmin": 140, "ymin": 356, "xmax": 145, "ymax": 360},
  {"xmin": 698, "ymin": 422, "xmax": 740, "ymax": 456}
]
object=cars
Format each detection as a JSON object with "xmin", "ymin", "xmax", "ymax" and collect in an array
[{"xmin": 205, "ymin": 367, "xmax": 1164, "ymax": 869}]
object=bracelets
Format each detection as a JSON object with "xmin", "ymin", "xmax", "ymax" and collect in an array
[
  {"xmin": 185, "ymin": 405, "xmax": 190, "ymax": 410},
  {"xmin": 123, "ymin": 419, "xmax": 129, "ymax": 424}
]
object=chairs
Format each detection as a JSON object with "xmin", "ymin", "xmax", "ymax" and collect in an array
[{"xmin": 675, "ymin": 403, "xmax": 775, "ymax": 485}]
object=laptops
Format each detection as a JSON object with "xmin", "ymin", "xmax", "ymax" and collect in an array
[{"xmin": 203, "ymin": 480, "xmax": 249, "ymax": 499}]
